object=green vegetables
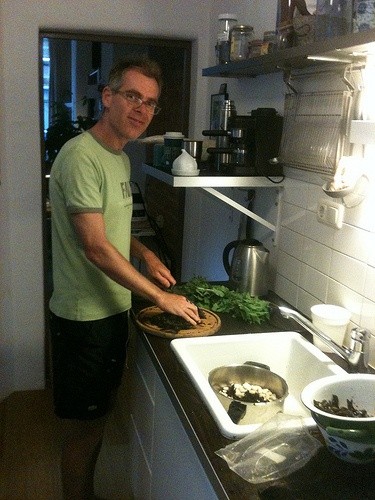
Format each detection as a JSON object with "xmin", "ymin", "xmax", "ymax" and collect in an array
[{"xmin": 161, "ymin": 274, "xmax": 271, "ymax": 325}]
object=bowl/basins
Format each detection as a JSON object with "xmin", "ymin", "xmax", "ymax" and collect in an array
[{"xmin": 299, "ymin": 372, "xmax": 375, "ymax": 463}]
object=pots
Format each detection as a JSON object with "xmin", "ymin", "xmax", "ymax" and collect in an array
[{"xmin": 207, "ymin": 360, "xmax": 288, "ymax": 425}]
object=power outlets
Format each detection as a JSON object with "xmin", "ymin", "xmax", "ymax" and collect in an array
[{"xmin": 317, "ymin": 199, "xmax": 344, "ymax": 230}]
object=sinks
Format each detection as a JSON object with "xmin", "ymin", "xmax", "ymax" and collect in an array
[{"xmin": 169, "ymin": 331, "xmax": 350, "ymax": 441}]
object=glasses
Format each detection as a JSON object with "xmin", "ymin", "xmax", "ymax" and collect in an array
[{"xmin": 112, "ymin": 89, "xmax": 161, "ymax": 115}]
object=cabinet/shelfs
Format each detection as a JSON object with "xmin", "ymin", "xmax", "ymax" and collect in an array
[
  {"xmin": 140, "ymin": 29, "xmax": 375, "ymax": 187},
  {"xmin": 126, "ymin": 338, "xmax": 219, "ymax": 500}
]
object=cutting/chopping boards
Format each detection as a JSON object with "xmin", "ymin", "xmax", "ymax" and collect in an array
[{"xmin": 135, "ymin": 304, "xmax": 222, "ymax": 338}]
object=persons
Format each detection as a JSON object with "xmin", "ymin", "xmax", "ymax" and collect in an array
[{"xmin": 49, "ymin": 53, "xmax": 202, "ymax": 500}]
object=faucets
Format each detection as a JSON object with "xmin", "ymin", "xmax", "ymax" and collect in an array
[{"xmin": 275, "ymin": 304, "xmax": 367, "ymax": 371}]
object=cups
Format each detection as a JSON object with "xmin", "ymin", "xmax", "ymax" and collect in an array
[
  {"xmin": 184, "ymin": 140, "xmax": 203, "ymax": 163},
  {"xmin": 163, "ymin": 131, "xmax": 184, "ymax": 166},
  {"xmin": 311, "ymin": 303, "xmax": 351, "ymax": 353}
]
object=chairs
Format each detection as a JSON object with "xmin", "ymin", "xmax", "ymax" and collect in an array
[{"xmin": 130, "ymin": 181, "xmax": 171, "ymax": 273}]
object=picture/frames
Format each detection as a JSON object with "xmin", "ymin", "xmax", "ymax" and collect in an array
[{"xmin": 210, "ymin": 94, "xmax": 228, "ymax": 139}]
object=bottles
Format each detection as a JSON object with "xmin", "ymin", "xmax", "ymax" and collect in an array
[
  {"xmin": 313, "ymin": 0, "xmax": 353, "ymax": 40},
  {"xmin": 352, "ymin": 0, "xmax": 375, "ymax": 34},
  {"xmin": 216, "ymin": 13, "xmax": 237, "ymax": 64},
  {"xmin": 230, "ymin": 24, "xmax": 256, "ymax": 61},
  {"xmin": 216, "ymin": 100, "xmax": 237, "ymax": 167},
  {"xmin": 248, "ymin": 31, "xmax": 278, "ymax": 59}
]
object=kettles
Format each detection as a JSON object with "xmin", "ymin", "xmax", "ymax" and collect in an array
[{"xmin": 222, "ymin": 238, "xmax": 271, "ymax": 300}]
